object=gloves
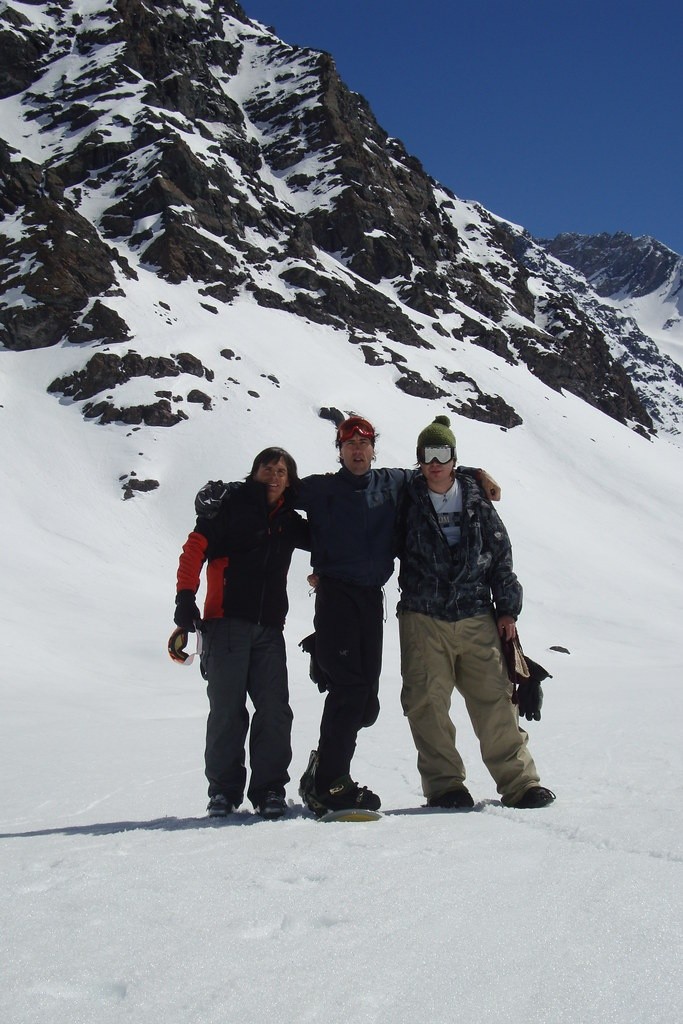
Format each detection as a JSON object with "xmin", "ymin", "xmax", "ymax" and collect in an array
[
  {"xmin": 195, "ymin": 480, "xmax": 230, "ymax": 519},
  {"xmin": 174, "ymin": 591, "xmax": 207, "ymax": 633},
  {"xmin": 512, "ymin": 657, "xmax": 552, "ymax": 721}
]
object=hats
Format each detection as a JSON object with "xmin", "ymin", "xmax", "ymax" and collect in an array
[{"xmin": 418, "ymin": 416, "xmax": 456, "ymax": 448}]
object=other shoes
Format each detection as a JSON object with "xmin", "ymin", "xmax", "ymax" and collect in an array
[
  {"xmin": 300, "ymin": 770, "xmax": 381, "ymax": 811},
  {"xmin": 428, "ymin": 791, "xmax": 474, "ymax": 809},
  {"xmin": 514, "ymin": 787, "xmax": 555, "ymax": 807}
]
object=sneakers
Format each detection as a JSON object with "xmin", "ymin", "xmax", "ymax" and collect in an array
[
  {"xmin": 253, "ymin": 791, "xmax": 287, "ymax": 819},
  {"xmin": 207, "ymin": 794, "xmax": 232, "ymax": 817}
]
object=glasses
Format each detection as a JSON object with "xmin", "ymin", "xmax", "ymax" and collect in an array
[
  {"xmin": 338, "ymin": 415, "xmax": 374, "ymax": 441},
  {"xmin": 416, "ymin": 445, "xmax": 452, "ymax": 465},
  {"xmin": 168, "ymin": 627, "xmax": 190, "ymax": 665}
]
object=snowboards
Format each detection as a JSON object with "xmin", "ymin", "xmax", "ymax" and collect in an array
[{"xmin": 312, "ymin": 806, "xmax": 384, "ymax": 822}]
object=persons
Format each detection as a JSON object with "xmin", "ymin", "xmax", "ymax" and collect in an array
[
  {"xmin": 306, "ymin": 415, "xmax": 556, "ymax": 809},
  {"xmin": 174, "ymin": 447, "xmax": 315, "ymax": 819},
  {"xmin": 194, "ymin": 415, "xmax": 501, "ymax": 817}
]
